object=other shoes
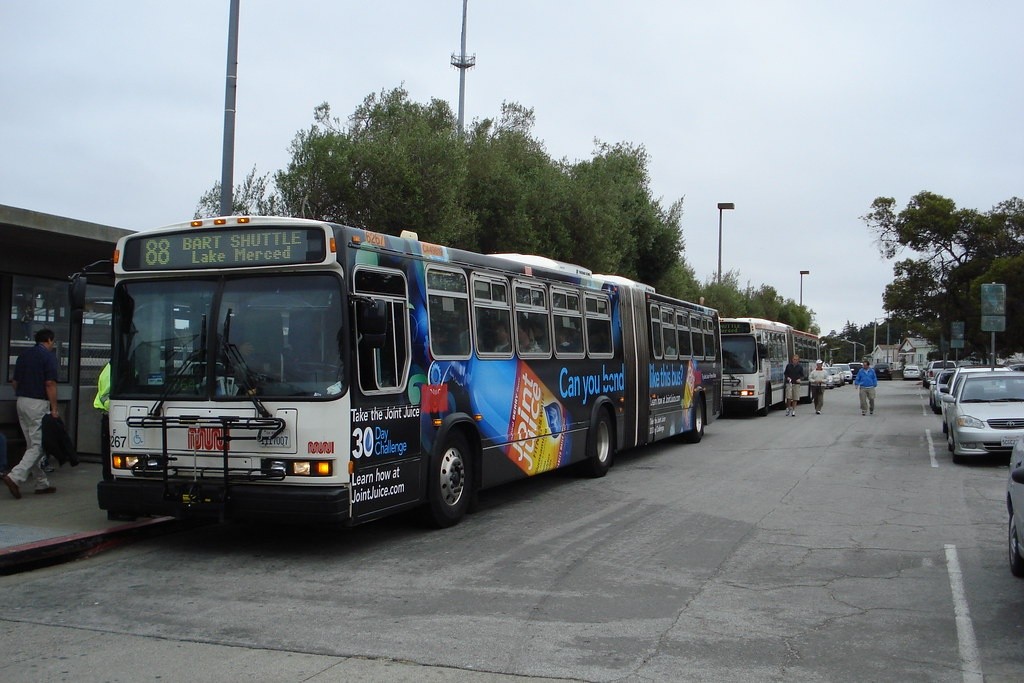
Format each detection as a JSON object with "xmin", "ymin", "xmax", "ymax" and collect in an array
[
  {"xmin": 786, "ymin": 408, "xmax": 790, "ymax": 416},
  {"xmin": 4, "ymin": 476, "xmax": 21, "ymax": 499},
  {"xmin": 816, "ymin": 411, "xmax": 821, "ymax": 414},
  {"xmin": 870, "ymin": 412, "xmax": 873, "ymax": 414},
  {"xmin": 36, "ymin": 487, "xmax": 56, "ymax": 494},
  {"xmin": 862, "ymin": 413, "xmax": 865, "ymax": 415},
  {"xmin": 792, "ymin": 411, "xmax": 795, "ymax": 416}
]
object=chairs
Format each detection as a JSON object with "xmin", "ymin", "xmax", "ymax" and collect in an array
[
  {"xmin": 965, "ymin": 384, "xmax": 984, "ymax": 399},
  {"xmin": 238, "ymin": 308, "xmax": 285, "ymax": 354},
  {"xmin": 288, "ymin": 310, "xmax": 335, "ymax": 361}
]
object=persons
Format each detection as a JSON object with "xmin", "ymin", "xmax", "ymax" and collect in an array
[
  {"xmin": 808, "ymin": 359, "xmax": 829, "ymax": 414},
  {"xmin": 491, "ymin": 313, "xmax": 582, "ymax": 355},
  {"xmin": 855, "ymin": 361, "xmax": 877, "ymax": 415},
  {"xmin": 734, "ymin": 351, "xmax": 754, "ymax": 368},
  {"xmin": 94, "ymin": 359, "xmax": 110, "ymax": 481},
  {"xmin": 784, "ymin": 355, "xmax": 805, "ymax": 416},
  {"xmin": 221, "ymin": 293, "xmax": 267, "ymax": 363},
  {"xmin": 664, "ymin": 336, "xmax": 677, "ymax": 355},
  {"xmin": 3, "ymin": 329, "xmax": 60, "ymax": 497}
]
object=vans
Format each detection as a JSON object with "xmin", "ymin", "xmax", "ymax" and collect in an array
[{"xmin": 848, "ymin": 362, "xmax": 863, "ymax": 380}]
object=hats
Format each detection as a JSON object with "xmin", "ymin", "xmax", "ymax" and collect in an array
[{"xmin": 816, "ymin": 359, "xmax": 823, "ymax": 364}]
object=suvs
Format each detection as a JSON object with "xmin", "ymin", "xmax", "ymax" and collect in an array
[
  {"xmin": 872, "ymin": 363, "xmax": 894, "ymax": 381},
  {"xmin": 831, "ymin": 364, "xmax": 855, "ymax": 384}
]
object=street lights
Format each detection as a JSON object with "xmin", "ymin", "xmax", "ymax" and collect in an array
[
  {"xmin": 717, "ymin": 202, "xmax": 735, "ymax": 284},
  {"xmin": 800, "ymin": 271, "xmax": 810, "ymax": 305}
]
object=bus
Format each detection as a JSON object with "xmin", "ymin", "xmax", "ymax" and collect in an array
[
  {"xmin": 720, "ymin": 317, "xmax": 821, "ymax": 417},
  {"xmin": 69, "ymin": 215, "xmax": 724, "ymax": 531}
]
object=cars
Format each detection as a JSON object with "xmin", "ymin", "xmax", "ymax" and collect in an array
[
  {"xmin": 903, "ymin": 366, "xmax": 921, "ymax": 380},
  {"xmin": 922, "ymin": 360, "xmax": 1024, "ymax": 436},
  {"xmin": 1006, "ymin": 434, "xmax": 1024, "ymax": 578},
  {"xmin": 822, "ymin": 368, "xmax": 836, "ymax": 389},
  {"xmin": 827, "ymin": 367, "xmax": 846, "ymax": 387},
  {"xmin": 942, "ymin": 370, "xmax": 1024, "ymax": 464}
]
